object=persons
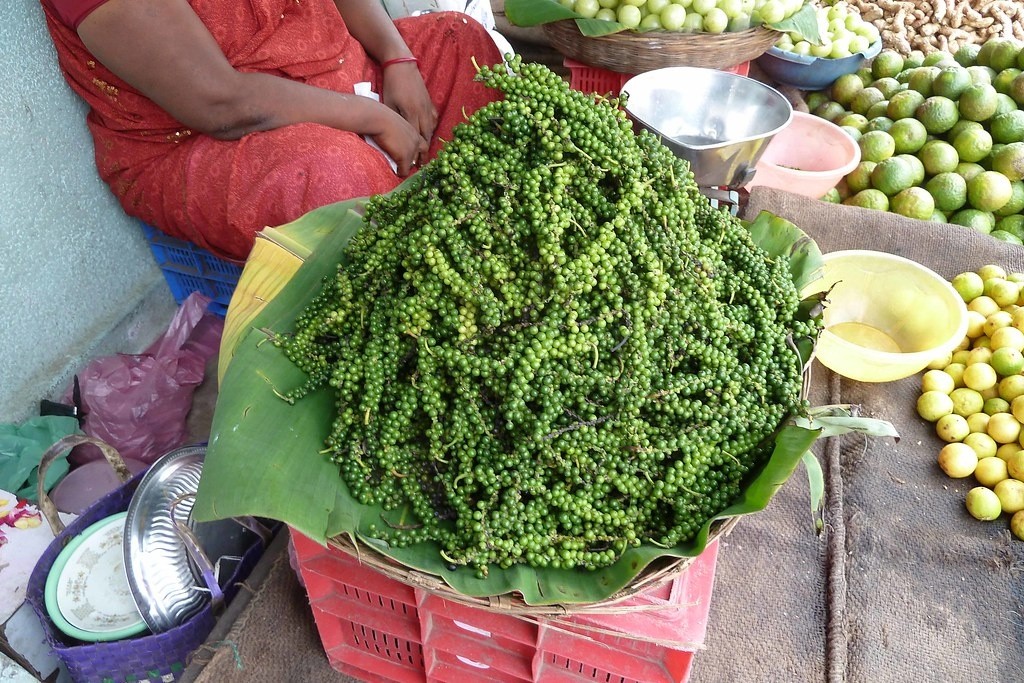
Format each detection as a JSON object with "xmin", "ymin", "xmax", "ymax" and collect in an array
[{"xmin": 39, "ymin": 1, "xmax": 511, "ymax": 268}]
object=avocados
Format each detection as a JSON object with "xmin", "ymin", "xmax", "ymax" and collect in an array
[{"xmin": 559, "ymin": 1, "xmax": 1024, "ymax": 540}]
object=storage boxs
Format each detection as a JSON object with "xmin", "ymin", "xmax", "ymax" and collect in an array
[
  {"xmin": 285, "ymin": 522, "xmax": 721, "ymax": 683},
  {"xmin": 138, "ymin": 219, "xmax": 242, "ymax": 315},
  {"xmin": 543, "ymin": 19, "xmax": 784, "ymax": 75},
  {"xmin": 564, "ymin": 58, "xmax": 752, "ymax": 114}
]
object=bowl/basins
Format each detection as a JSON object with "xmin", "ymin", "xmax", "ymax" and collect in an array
[
  {"xmin": 48, "ymin": 456, "xmax": 152, "ymax": 515},
  {"xmin": 122, "ymin": 444, "xmax": 281, "ymax": 637},
  {"xmin": 799, "ymin": 250, "xmax": 969, "ymax": 383},
  {"xmin": 618, "ymin": 66, "xmax": 795, "ymax": 187},
  {"xmin": 744, "ymin": 110, "xmax": 862, "ymax": 199},
  {"xmin": 756, "ymin": 33, "xmax": 883, "ymax": 91}
]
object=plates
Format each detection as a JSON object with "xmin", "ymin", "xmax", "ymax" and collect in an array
[{"xmin": 43, "ymin": 511, "xmax": 149, "ymax": 643}]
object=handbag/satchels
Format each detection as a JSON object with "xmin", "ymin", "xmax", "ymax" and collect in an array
[{"xmin": 25, "ymin": 436, "xmax": 280, "ymax": 683}]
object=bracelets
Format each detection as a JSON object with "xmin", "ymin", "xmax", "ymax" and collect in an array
[{"xmin": 381, "ymin": 57, "xmax": 418, "ymax": 69}]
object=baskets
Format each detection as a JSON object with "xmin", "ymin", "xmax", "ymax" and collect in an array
[
  {"xmin": 133, "ymin": 216, "xmax": 243, "ymax": 316},
  {"xmin": 542, "ymin": 19, "xmax": 786, "ymax": 74},
  {"xmin": 564, "ymin": 58, "xmax": 749, "ymax": 106},
  {"xmin": 284, "ymin": 526, "xmax": 718, "ymax": 679}
]
